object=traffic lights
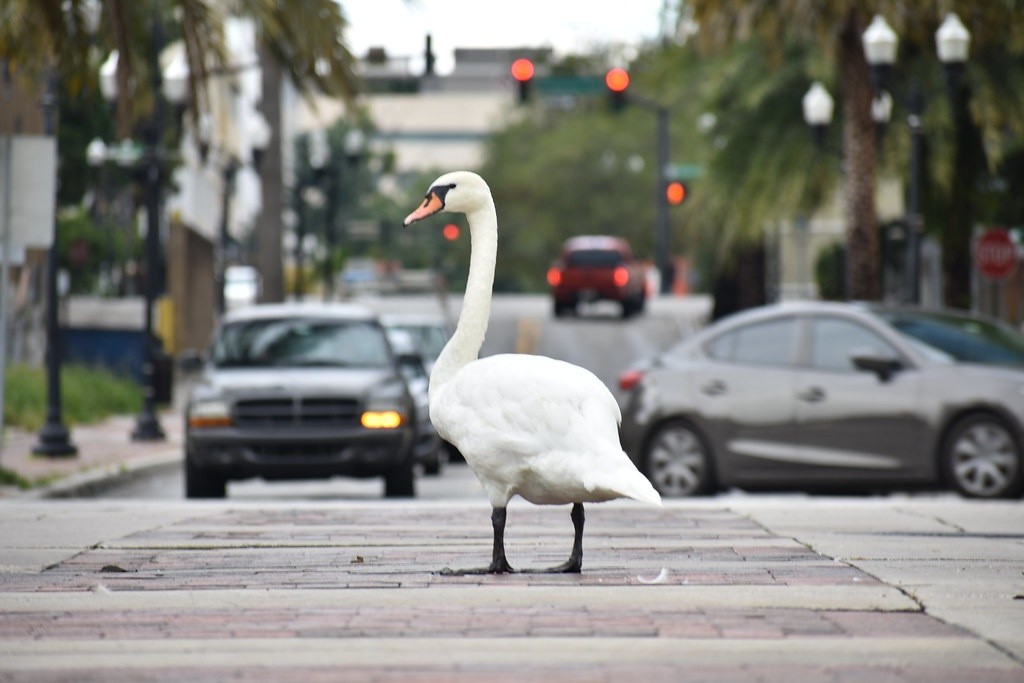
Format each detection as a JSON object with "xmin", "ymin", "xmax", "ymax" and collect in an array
[
  {"xmin": 513, "ymin": 57, "xmax": 531, "ymax": 100},
  {"xmin": 607, "ymin": 68, "xmax": 629, "ymax": 112},
  {"xmin": 662, "ymin": 176, "xmax": 688, "ymax": 208}
]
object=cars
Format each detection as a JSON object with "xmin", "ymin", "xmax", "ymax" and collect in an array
[
  {"xmin": 179, "ymin": 302, "xmax": 420, "ymax": 504},
  {"xmin": 547, "ymin": 232, "xmax": 645, "ymax": 314},
  {"xmin": 382, "ymin": 316, "xmax": 476, "ymax": 475},
  {"xmin": 610, "ymin": 295, "xmax": 1024, "ymax": 501}
]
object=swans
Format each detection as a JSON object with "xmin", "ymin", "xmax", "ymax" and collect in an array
[{"xmin": 402, "ymin": 171, "xmax": 664, "ymax": 574}]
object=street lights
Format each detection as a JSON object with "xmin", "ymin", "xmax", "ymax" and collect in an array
[
  {"xmin": 865, "ymin": 10, "xmax": 975, "ymax": 302},
  {"xmin": 809, "ymin": 68, "xmax": 900, "ymax": 300}
]
